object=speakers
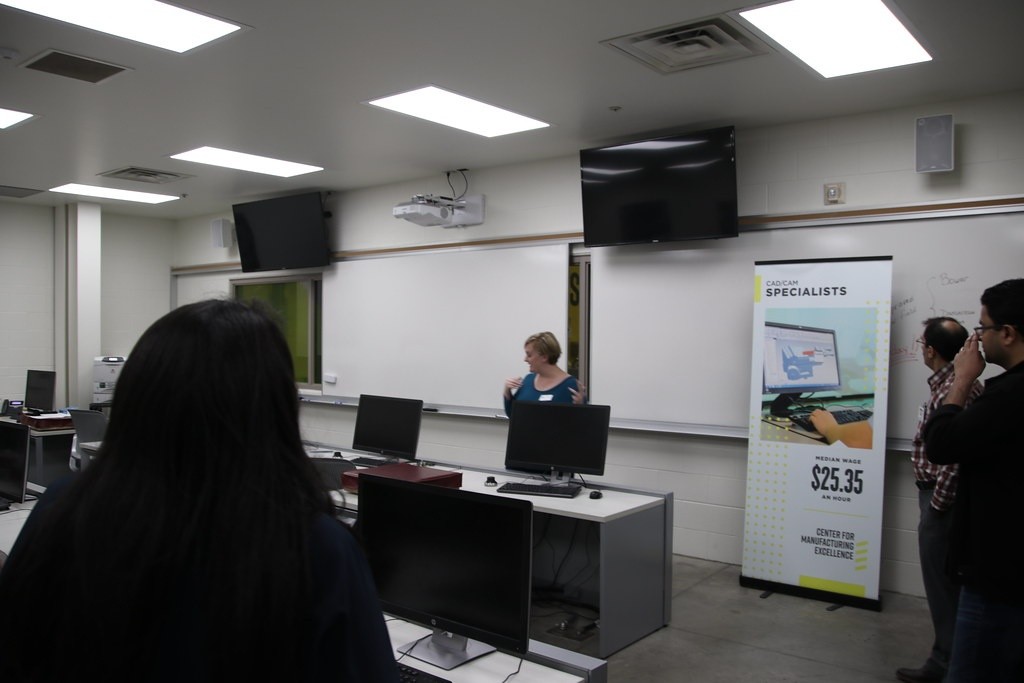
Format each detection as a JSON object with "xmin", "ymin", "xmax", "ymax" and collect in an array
[
  {"xmin": 914, "ymin": 113, "xmax": 954, "ymax": 174},
  {"xmin": 211, "ymin": 218, "xmax": 233, "ymax": 248}
]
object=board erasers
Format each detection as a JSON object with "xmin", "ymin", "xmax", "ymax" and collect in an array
[{"xmin": 324, "ymin": 375, "xmax": 337, "ymax": 384}]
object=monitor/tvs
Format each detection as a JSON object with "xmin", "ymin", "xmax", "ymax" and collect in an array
[
  {"xmin": 351, "ymin": 394, "xmax": 423, "ymax": 461},
  {"xmin": 357, "ymin": 474, "xmax": 533, "ymax": 672},
  {"xmin": 762, "ymin": 321, "xmax": 841, "ymax": 418},
  {"xmin": 395, "ymin": 661, "xmax": 453, "ymax": 683},
  {"xmin": 25, "ymin": 370, "xmax": 56, "ymax": 413},
  {"xmin": 580, "ymin": 126, "xmax": 737, "ymax": 248},
  {"xmin": 0, "ymin": 421, "xmax": 31, "ymax": 511},
  {"xmin": 232, "ymin": 191, "xmax": 331, "ymax": 272},
  {"xmin": 505, "ymin": 400, "xmax": 610, "ymax": 486}
]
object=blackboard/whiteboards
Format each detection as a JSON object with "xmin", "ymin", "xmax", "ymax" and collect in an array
[
  {"xmin": 321, "ymin": 241, "xmax": 569, "ymax": 410},
  {"xmin": 588, "ymin": 212, "xmax": 1024, "ymax": 441}
]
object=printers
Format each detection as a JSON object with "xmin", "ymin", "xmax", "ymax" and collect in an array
[{"xmin": 92, "ymin": 357, "xmax": 127, "ymax": 418}]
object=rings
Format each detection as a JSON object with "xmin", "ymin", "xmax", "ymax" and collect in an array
[{"xmin": 959, "ymin": 348, "xmax": 964, "ymax": 352}]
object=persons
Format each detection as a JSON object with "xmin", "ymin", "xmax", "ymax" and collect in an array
[
  {"xmin": 923, "ymin": 279, "xmax": 1024, "ymax": 683},
  {"xmin": 897, "ymin": 316, "xmax": 986, "ymax": 683},
  {"xmin": 0, "ymin": 298, "xmax": 401, "ymax": 683},
  {"xmin": 504, "ymin": 331, "xmax": 586, "ymax": 477}
]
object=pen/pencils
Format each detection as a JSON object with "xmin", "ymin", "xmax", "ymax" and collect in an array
[
  {"xmin": 334, "ymin": 401, "xmax": 342, "ymax": 404},
  {"xmin": 423, "ymin": 408, "xmax": 438, "ymax": 412},
  {"xmin": 495, "ymin": 414, "xmax": 509, "ymax": 419},
  {"xmin": 300, "ymin": 399, "xmax": 310, "ymax": 402}
]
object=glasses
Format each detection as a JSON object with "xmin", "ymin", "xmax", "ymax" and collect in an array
[
  {"xmin": 915, "ymin": 338, "xmax": 933, "ymax": 349},
  {"xmin": 974, "ymin": 326, "xmax": 1005, "ymax": 340}
]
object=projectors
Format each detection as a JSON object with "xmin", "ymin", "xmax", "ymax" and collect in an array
[{"xmin": 393, "ymin": 204, "xmax": 452, "ymax": 227}]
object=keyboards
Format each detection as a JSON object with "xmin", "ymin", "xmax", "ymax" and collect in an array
[
  {"xmin": 788, "ymin": 409, "xmax": 873, "ymax": 431},
  {"xmin": 350, "ymin": 457, "xmax": 397, "ymax": 466},
  {"xmin": 497, "ymin": 482, "xmax": 581, "ymax": 498}
]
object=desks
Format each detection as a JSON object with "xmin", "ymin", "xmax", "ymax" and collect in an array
[
  {"xmin": 384, "ymin": 612, "xmax": 608, "ymax": 683},
  {"xmin": 301, "ymin": 439, "xmax": 675, "ymax": 658},
  {"xmin": 0, "ymin": 415, "xmax": 101, "ymax": 497}
]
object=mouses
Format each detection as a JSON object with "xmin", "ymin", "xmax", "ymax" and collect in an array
[{"xmin": 589, "ymin": 491, "xmax": 602, "ymax": 499}]
